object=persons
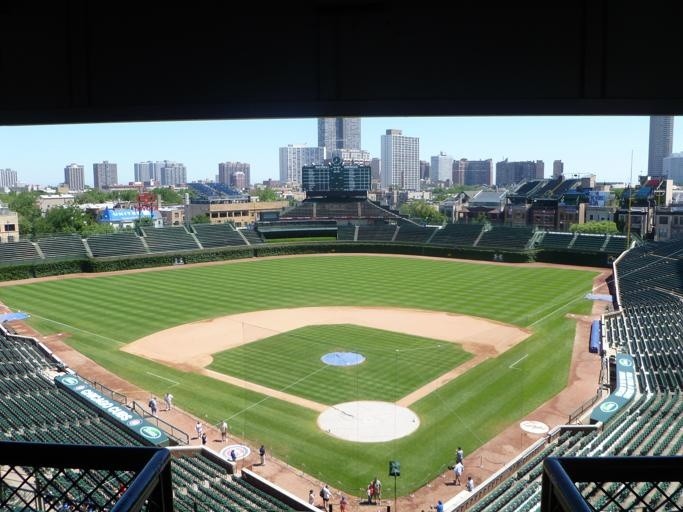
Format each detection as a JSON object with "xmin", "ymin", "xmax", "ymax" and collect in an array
[
  {"xmin": 466, "ymin": 476, "xmax": 473, "ymax": 492},
  {"xmin": 150, "ymin": 397, "xmax": 157, "ymax": 415},
  {"xmin": 163, "ymin": 392, "xmax": 173, "ymax": 410},
  {"xmin": 258, "ymin": 444, "xmax": 265, "ymax": 465},
  {"xmin": 219, "ymin": 419, "xmax": 228, "ymax": 443},
  {"xmin": 435, "ymin": 501, "xmax": 443, "ymax": 512},
  {"xmin": 201, "ymin": 433, "xmax": 208, "ymax": 446},
  {"xmin": 456, "ymin": 446, "xmax": 464, "ymax": 466},
  {"xmin": 308, "ymin": 476, "xmax": 383, "ymax": 512},
  {"xmin": 230, "ymin": 450, "xmax": 236, "ymax": 461},
  {"xmin": 195, "ymin": 422, "xmax": 201, "ymax": 439},
  {"xmin": 453, "ymin": 460, "xmax": 465, "ymax": 486}
]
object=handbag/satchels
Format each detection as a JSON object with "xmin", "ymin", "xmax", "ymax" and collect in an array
[{"xmin": 319, "ymin": 489, "xmax": 324, "ymax": 498}]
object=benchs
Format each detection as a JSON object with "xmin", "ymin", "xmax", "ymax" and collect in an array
[
  {"xmin": 0, "ymin": 329, "xmax": 300, "ymax": 512},
  {"xmin": 337, "ymin": 223, "xmax": 683, "ymax": 512},
  {"xmin": 0, "ymin": 224, "xmax": 264, "ymax": 262}
]
